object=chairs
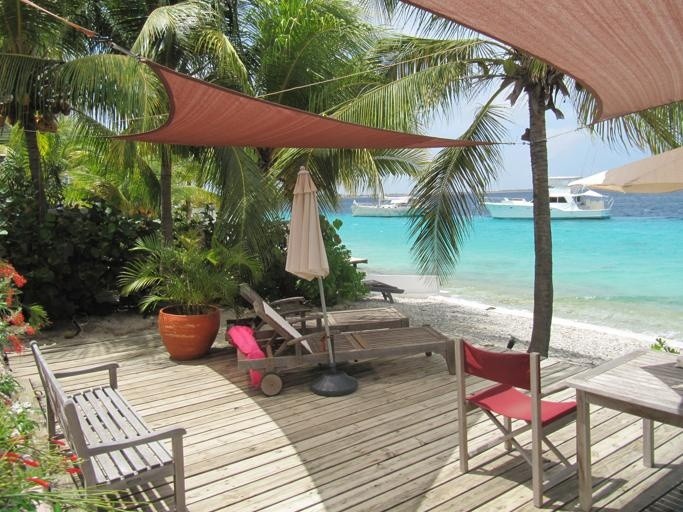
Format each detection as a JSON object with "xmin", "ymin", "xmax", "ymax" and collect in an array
[
  {"xmin": 453, "ymin": 337, "xmax": 576, "ymax": 509},
  {"xmin": 226, "ymin": 280, "xmax": 457, "ymax": 401}
]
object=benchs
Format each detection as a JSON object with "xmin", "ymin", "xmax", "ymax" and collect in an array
[{"xmin": 28, "ymin": 335, "xmax": 185, "ymax": 511}]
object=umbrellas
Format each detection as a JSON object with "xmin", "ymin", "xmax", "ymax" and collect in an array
[
  {"xmin": 568, "ymin": 145, "xmax": 683, "ymax": 193},
  {"xmin": 285, "ymin": 165, "xmax": 335, "ymax": 363}
]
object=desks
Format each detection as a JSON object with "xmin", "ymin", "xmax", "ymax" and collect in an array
[{"xmin": 563, "ymin": 347, "xmax": 683, "ymax": 511}]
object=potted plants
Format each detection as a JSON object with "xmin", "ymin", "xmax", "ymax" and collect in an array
[{"xmin": 115, "ymin": 226, "xmax": 264, "ymax": 361}]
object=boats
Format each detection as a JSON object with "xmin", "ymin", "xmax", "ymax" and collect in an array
[
  {"xmin": 479, "ymin": 176, "xmax": 614, "ymax": 218},
  {"xmin": 351, "ymin": 196, "xmax": 428, "ymax": 217}
]
